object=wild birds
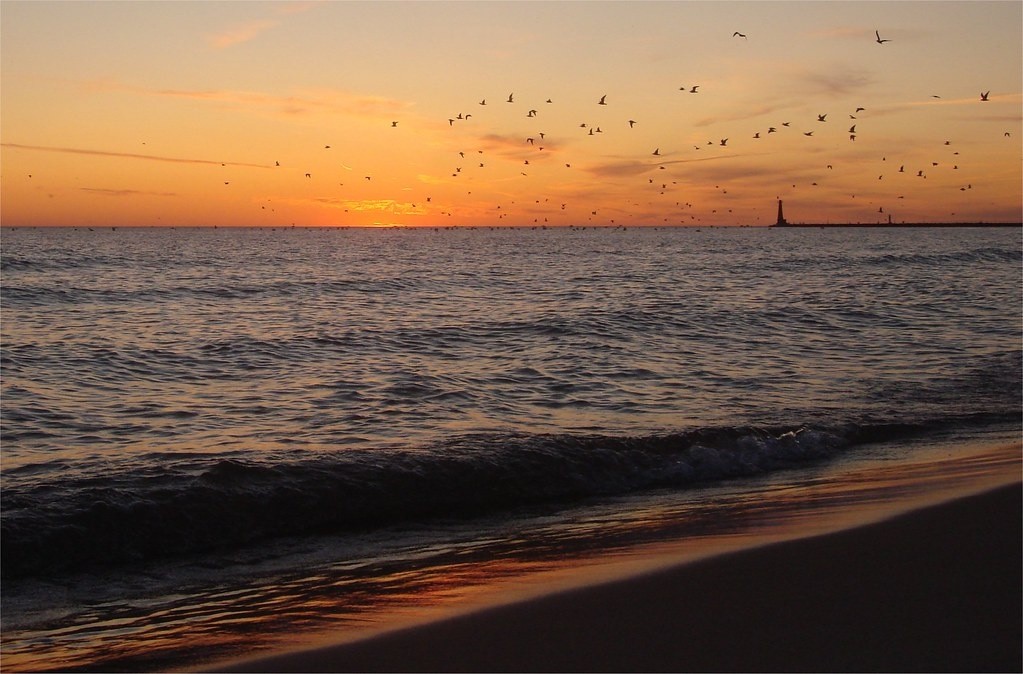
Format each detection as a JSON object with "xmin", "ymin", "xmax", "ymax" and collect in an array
[
  {"xmin": 782, "ymin": 120, "xmax": 791, "ymax": 128},
  {"xmin": 944, "ymin": 140, "xmax": 950, "ymax": 146},
  {"xmin": 678, "ymin": 85, "xmax": 701, "ymax": 95},
  {"xmin": 802, "ymin": 131, "xmax": 814, "ymax": 137},
  {"xmin": 1005, "ymin": 132, "xmax": 1010, "ymax": 137},
  {"xmin": 693, "ymin": 137, "xmax": 729, "ymax": 149},
  {"xmin": 930, "ymin": 95, "xmax": 941, "ymax": 99},
  {"xmin": 959, "ymin": 184, "xmax": 972, "ymax": 191},
  {"xmin": 953, "ymin": 165, "xmax": 958, "ymax": 169},
  {"xmin": 733, "ymin": 31, "xmax": 747, "ymax": 40},
  {"xmin": 979, "ymin": 91, "xmax": 990, "ymax": 101},
  {"xmin": 752, "ymin": 132, "xmax": 761, "ymax": 139},
  {"xmin": 875, "ymin": 30, "xmax": 892, "ymax": 44},
  {"xmin": 7, "ymin": 157, "xmax": 956, "ymax": 235},
  {"xmin": 767, "ymin": 127, "xmax": 777, "ymax": 134},
  {"xmin": 652, "ymin": 148, "xmax": 662, "ymax": 156},
  {"xmin": 817, "ymin": 114, "xmax": 828, "ymax": 122},
  {"xmin": 847, "ymin": 107, "xmax": 866, "ymax": 141},
  {"xmin": 136, "ymin": 75, "xmax": 638, "ymax": 159},
  {"xmin": 953, "ymin": 151, "xmax": 959, "ymax": 155}
]
